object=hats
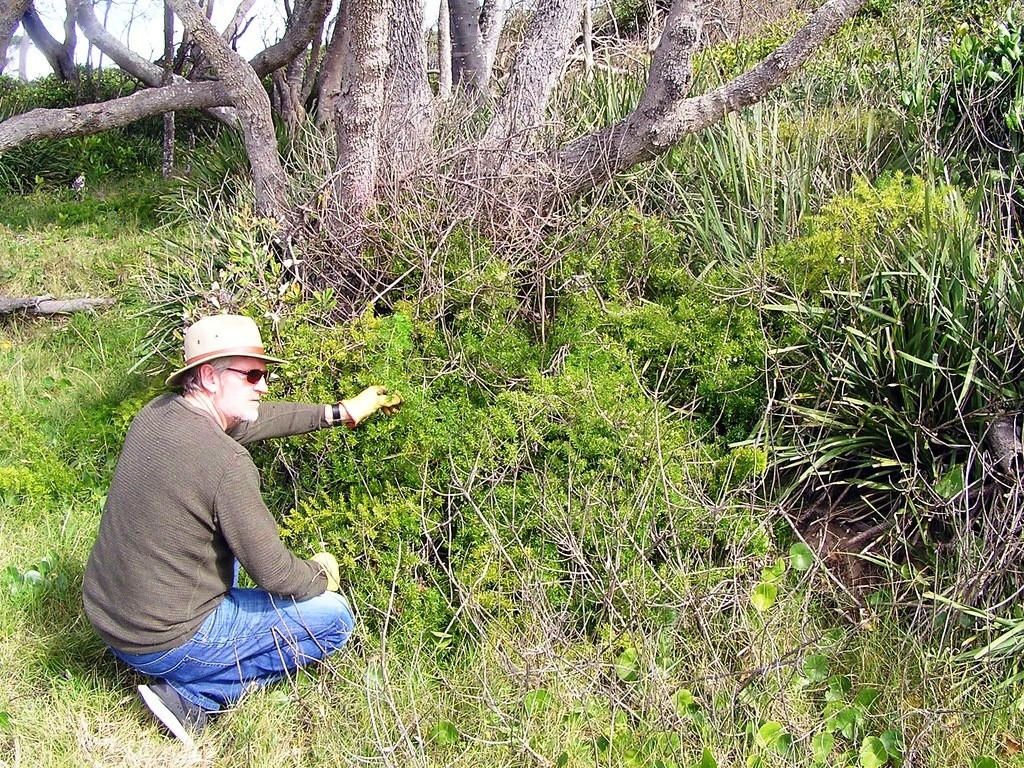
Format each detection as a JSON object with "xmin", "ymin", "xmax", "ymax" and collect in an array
[{"xmin": 165, "ymin": 315, "xmax": 288, "ymax": 387}]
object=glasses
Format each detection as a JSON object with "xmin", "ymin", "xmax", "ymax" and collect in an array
[{"xmin": 221, "ymin": 368, "xmax": 270, "ymax": 385}]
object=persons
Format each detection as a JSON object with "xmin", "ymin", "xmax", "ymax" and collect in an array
[{"xmin": 81, "ymin": 314, "xmax": 404, "ymax": 746}]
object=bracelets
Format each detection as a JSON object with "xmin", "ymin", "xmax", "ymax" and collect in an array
[
  {"xmin": 331, "ymin": 403, "xmax": 342, "ymax": 427},
  {"xmin": 338, "ymin": 402, "xmax": 356, "ymax": 428}
]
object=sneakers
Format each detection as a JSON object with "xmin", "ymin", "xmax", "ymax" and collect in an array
[{"xmin": 138, "ymin": 677, "xmax": 208, "ymax": 752}]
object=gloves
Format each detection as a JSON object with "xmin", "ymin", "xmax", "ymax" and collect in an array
[
  {"xmin": 310, "ymin": 553, "xmax": 340, "ymax": 591},
  {"xmin": 339, "ymin": 386, "xmax": 405, "ymax": 427}
]
